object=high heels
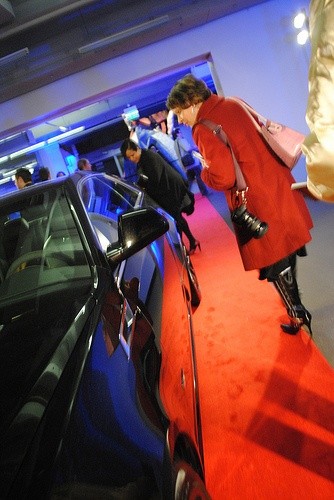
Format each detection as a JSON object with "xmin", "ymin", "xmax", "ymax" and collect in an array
[{"xmin": 186, "ymin": 240, "xmax": 201, "ymax": 255}]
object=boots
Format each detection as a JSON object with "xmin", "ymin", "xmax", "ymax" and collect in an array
[{"xmin": 272, "ymin": 265, "xmax": 313, "ymax": 335}]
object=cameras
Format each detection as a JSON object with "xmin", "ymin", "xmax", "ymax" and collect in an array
[{"xmin": 231, "ymin": 204, "xmax": 268, "ymax": 239}]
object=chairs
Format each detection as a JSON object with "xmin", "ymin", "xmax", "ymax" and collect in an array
[{"xmin": 43, "ymin": 199, "xmax": 89, "ymax": 269}]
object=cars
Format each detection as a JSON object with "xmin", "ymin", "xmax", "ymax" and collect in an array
[{"xmin": 0, "ymin": 169, "xmax": 212, "ymax": 500}]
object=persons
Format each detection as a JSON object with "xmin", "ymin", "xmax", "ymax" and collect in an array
[
  {"xmin": 166, "ymin": 73, "xmax": 314, "ymax": 336},
  {"xmin": 100, "ymin": 277, "xmax": 162, "ymax": 364},
  {"xmin": 14, "ymin": 127, "xmax": 213, "ymax": 255},
  {"xmin": 300, "ymin": 0, "xmax": 334, "ymax": 203}
]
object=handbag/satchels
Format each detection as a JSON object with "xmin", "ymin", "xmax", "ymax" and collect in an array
[
  {"xmin": 181, "ymin": 190, "xmax": 194, "ymax": 215},
  {"xmin": 225, "ymin": 95, "xmax": 306, "ymax": 171},
  {"xmin": 182, "ymin": 154, "xmax": 194, "ymax": 167}
]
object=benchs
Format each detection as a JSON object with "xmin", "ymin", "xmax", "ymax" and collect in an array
[{"xmin": 4, "ymin": 215, "xmax": 45, "ymax": 258}]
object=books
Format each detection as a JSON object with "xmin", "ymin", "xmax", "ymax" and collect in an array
[{"xmin": 291, "ymin": 182, "xmax": 319, "ymax": 201}]
